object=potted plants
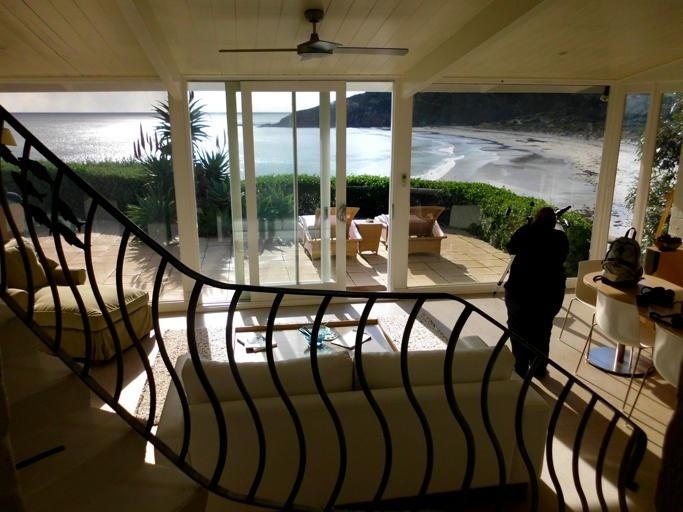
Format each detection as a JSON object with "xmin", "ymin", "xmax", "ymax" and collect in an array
[{"xmin": 125, "ymin": 122, "xmax": 180, "ymax": 261}]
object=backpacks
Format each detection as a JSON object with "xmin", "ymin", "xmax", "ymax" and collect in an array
[{"xmin": 592, "ymin": 225, "xmax": 645, "ymax": 290}]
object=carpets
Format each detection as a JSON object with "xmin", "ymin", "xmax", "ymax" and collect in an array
[{"xmin": 134, "ymin": 305, "xmax": 448, "ymax": 425}]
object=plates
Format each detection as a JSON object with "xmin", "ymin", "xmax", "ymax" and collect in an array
[{"xmin": 321, "ymin": 330, "xmax": 339, "ymax": 340}]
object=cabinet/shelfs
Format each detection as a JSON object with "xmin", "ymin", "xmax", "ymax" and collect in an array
[{"xmin": 644, "ymin": 246, "xmax": 683, "ymax": 287}]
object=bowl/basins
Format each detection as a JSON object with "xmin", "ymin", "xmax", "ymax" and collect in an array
[
  {"xmin": 304, "ymin": 333, "xmax": 325, "ymax": 346},
  {"xmin": 650, "ymin": 237, "xmax": 683, "ymax": 253}
]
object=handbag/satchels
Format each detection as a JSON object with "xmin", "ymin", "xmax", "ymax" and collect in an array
[{"xmin": 635, "ymin": 285, "xmax": 676, "ymax": 309}]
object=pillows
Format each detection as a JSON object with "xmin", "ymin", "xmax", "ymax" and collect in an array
[{"xmin": 4, "ymin": 235, "xmax": 47, "ymax": 292}]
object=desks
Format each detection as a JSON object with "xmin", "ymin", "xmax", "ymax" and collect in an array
[{"xmin": 582, "ymin": 270, "xmax": 683, "ymax": 378}]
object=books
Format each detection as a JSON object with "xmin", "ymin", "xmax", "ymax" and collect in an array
[
  {"xmin": 244, "ymin": 330, "xmax": 266, "ymax": 350},
  {"xmin": 235, "ymin": 333, "xmax": 277, "ymax": 354},
  {"xmin": 303, "ymin": 325, "xmax": 339, "ymax": 341},
  {"xmin": 330, "ymin": 327, "xmax": 370, "ymax": 351},
  {"xmin": 298, "ymin": 328, "xmax": 339, "ymax": 339}
]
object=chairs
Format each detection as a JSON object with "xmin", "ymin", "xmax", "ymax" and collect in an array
[
  {"xmin": 559, "ymin": 259, "xmax": 604, "ymax": 363},
  {"xmin": 2, "ymin": 240, "xmax": 154, "ymax": 361},
  {"xmin": 298, "ymin": 205, "xmax": 363, "ymax": 261},
  {"xmin": 373, "ymin": 204, "xmax": 447, "ymax": 262},
  {"xmin": 574, "ymin": 291, "xmax": 654, "ymax": 411},
  {"xmin": 625, "ymin": 310, "xmax": 683, "ymax": 424}
]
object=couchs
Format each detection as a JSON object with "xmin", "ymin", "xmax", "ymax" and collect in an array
[{"xmin": 155, "ymin": 334, "xmax": 551, "ymax": 512}]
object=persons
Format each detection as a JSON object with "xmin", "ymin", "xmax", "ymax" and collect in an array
[{"xmin": 501, "ymin": 206, "xmax": 570, "ymax": 379}]
